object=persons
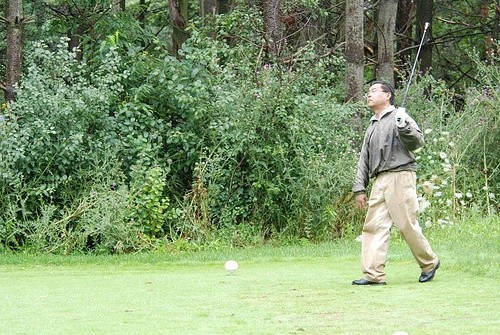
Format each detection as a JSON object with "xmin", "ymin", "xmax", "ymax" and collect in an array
[{"xmin": 351, "ymin": 81, "xmax": 441, "ymax": 286}]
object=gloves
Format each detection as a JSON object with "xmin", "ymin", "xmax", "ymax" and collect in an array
[{"xmin": 394, "ymin": 107, "xmax": 407, "ymax": 129}]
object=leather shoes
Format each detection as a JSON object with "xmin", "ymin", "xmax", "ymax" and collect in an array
[
  {"xmin": 352, "ymin": 278, "xmax": 387, "ymax": 286},
  {"xmin": 418, "ymin": 259, "xmax": 440, "ymax": 283}
]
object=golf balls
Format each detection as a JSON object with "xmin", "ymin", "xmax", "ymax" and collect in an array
[{"xmin": 224, "ymin": 261, "xmax": 238, "ymax": 270}]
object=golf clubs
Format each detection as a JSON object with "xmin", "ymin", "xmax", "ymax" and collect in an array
[{"xmin": 396, "ymin": 21, "xmax": 431, "ymax": 122}]
